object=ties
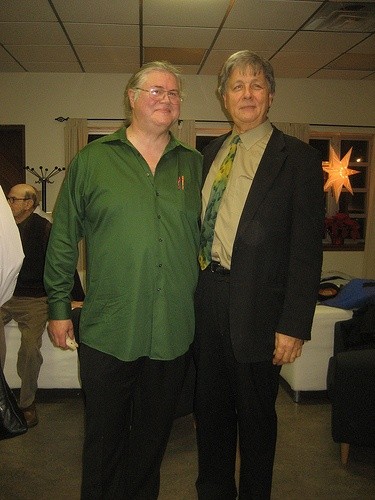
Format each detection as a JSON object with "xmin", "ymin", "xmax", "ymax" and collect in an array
[{"xmin": 198, "ymin": 135, "xmax": 240, "ymax": 271}]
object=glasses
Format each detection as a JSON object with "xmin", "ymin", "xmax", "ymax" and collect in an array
[
  {"xmin": 135, "ymin": 87, "xmax": 184, "ymax": 105},
  {"xmin": 7, "ymin": 197, "xmax": 32, "ymax": 204}
]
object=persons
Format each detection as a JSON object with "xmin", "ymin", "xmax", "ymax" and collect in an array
[
  {"xmin": 191, "ymin": 50, "xmax": 323, "ymax": 500},
  {"xmin": 0, "ymin": 183, "xmax": 86, "ymax": 439},
  {"xmin": 43, "ymin": 61, "xmax": 204, "ymax": 500}
]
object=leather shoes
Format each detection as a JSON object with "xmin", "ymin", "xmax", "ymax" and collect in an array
[{"xmin": 22, "ymin": 402, "xmax": 39, "ymax": 427}]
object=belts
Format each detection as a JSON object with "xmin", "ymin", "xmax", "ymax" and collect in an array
[{"xmin": 207, "ymin": 261, "xmax": 229, "ymax": 274}]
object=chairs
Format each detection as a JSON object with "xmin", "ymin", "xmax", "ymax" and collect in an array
[{"xmin": 327, "ymin": 302, "xmax": 375, "ymax": 463}]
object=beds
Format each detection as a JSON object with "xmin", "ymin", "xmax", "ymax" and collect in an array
[
  {"xmin": 282, "ymin": 272, "xmax": 354, "ymax": 403},
  {"xmin": 1, "ymin": 312, "xmax": 93, "ymax": 397}
]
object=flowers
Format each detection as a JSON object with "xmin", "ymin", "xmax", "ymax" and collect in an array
[{"xmin": 322, "ymin": 212, "xmax": 361, "ymax": 240}]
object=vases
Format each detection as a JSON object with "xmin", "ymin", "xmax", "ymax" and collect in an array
[{"xmin": 331, "ymin": 229, "xmax": 344, "ymax": 245}]
